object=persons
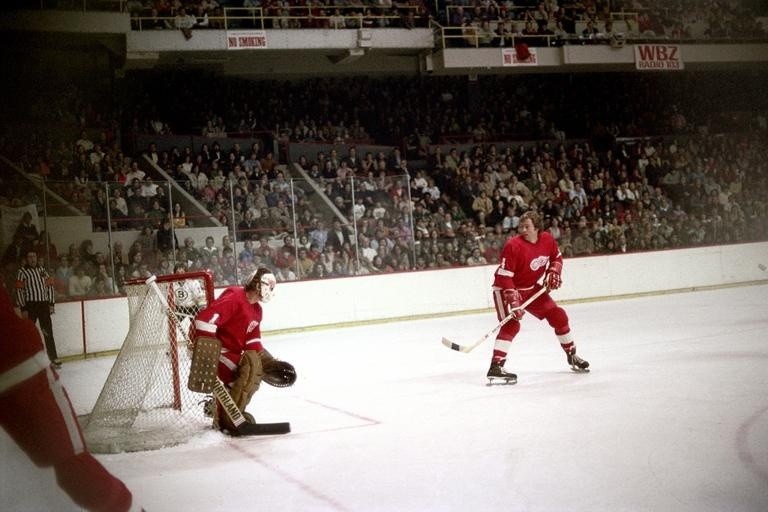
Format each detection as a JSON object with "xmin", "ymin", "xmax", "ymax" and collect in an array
[
  {"xmin": 187, "ymin": 266, "xmax": 297, "ymax": 438},
  {"xmin": 1, "ymin": 0, "xmax": 767, "ymax": 307},
  {"xmin": 484, "ymin": 210, "xmax": 589, "ymax": 379},
  {"xmin": 165, "ymin": 263, "xmax": 208, "ymax": 357},
  {"xmin": 0, "ymin": 280, "xmax": 146, "ymax": 511},
  {"xmin": 15, "ymin": 250, "xmax": 63, "ymax": 366}
]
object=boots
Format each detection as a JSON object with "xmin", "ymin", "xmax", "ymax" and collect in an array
[
  {"xmin": 566, "ymin": 346, "xmax": 589, "ymax": 368},
  {"xmin": 487, "ymin": 359, "xmax": 516, "ymax": 377}
]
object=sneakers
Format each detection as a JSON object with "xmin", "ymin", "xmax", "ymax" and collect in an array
[
  {"xmin": 50, "ymin": 357, "xmax": 61, "ymax": 365},
  {"xmin": 55, "ymin": 451, "xmax": 132, "ymax": 512}
]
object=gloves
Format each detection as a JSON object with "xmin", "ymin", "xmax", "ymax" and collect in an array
[
  {"xmin": 503, "ymin": 289, "xmax": 525, "ymax": 321},
  {"xmin": 543, "ymin": 262, "xmax": 562, "ymax": 289}
]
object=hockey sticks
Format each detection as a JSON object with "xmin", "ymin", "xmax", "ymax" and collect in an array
[
  {"xmin": 144, "ymin": 274, "xmax": 290, "ymax": 436},
  {"xmin": 441, "ymin": 286, "xmax": 546, "ymax": 353}
]
